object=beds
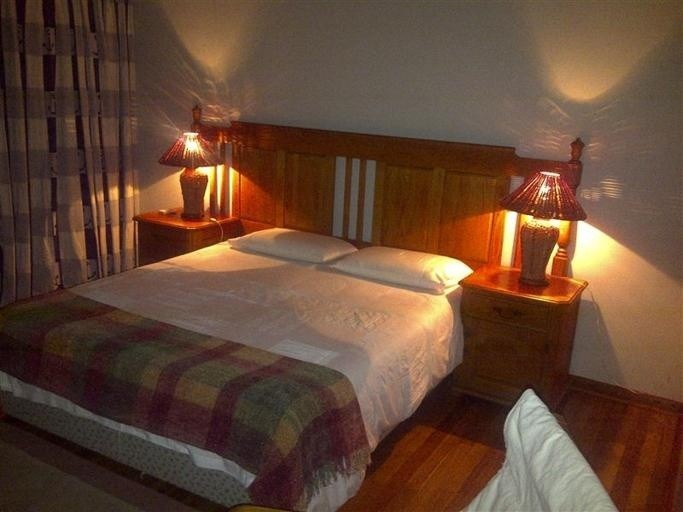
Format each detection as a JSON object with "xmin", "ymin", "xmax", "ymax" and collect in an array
[{"xmin": 0, "ymin": 108, "xmax": 584, "ymax": 511}]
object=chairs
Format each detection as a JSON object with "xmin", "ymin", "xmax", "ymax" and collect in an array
[{"xmin": 455, "ymin": 386, "xmax": 617, "ymax": 510}]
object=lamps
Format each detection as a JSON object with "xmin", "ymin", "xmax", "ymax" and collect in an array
[
  {"xmin": 497, "ymin": 172, "xmax": 588, "ymax": 288},
  {"xmin": 157, "ymin": 132, "xmax": 226, "ymax": 221}
]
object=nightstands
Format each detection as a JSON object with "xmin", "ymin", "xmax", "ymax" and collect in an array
[
  {"xmin": 132, "ymin": 209, "xmax": 242, "ymax": 269},
  {"xmin": 449, "ymin": 262, "xmax": 589, "ymax": 416}
]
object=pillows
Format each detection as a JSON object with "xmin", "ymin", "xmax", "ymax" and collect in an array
[
  {"xmin": 327, "ymin": 241, "xmax": 476, "ymax": 293},
  {"xmin": 226, "ymin": 224, "xmax": 359, "ymax": 266}
]
